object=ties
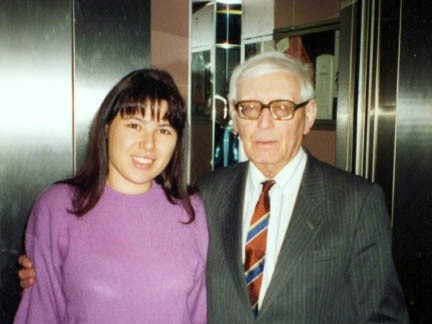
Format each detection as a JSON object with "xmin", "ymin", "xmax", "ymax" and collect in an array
[{"xmin": 243, "ymin": 180, "xmax": 276, "ymax": 320}]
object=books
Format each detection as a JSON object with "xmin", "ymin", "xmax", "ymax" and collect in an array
[
  {"xmin": 333, "ymin": 30, "xmax": 339, "ymax": 100},
  {"xmin": 290, "ymin": 36, "xmax": 316, "ymax": 81}
]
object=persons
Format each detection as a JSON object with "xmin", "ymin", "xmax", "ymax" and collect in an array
[
  {"xmin": 18, "ymin": 52, "xmax": 409, "ymax": 324},
  {"xmin": 14, "ymin": 68, "xmax": 209, "ymax": 324},
  {"xmin": 205, "ymin": 94, "xmax": 238, "ymax": 171}
]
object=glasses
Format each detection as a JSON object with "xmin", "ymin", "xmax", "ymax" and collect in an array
[{"xmin": 235, "ymin": 100, "xmax": 309, "ymax": 120}]
